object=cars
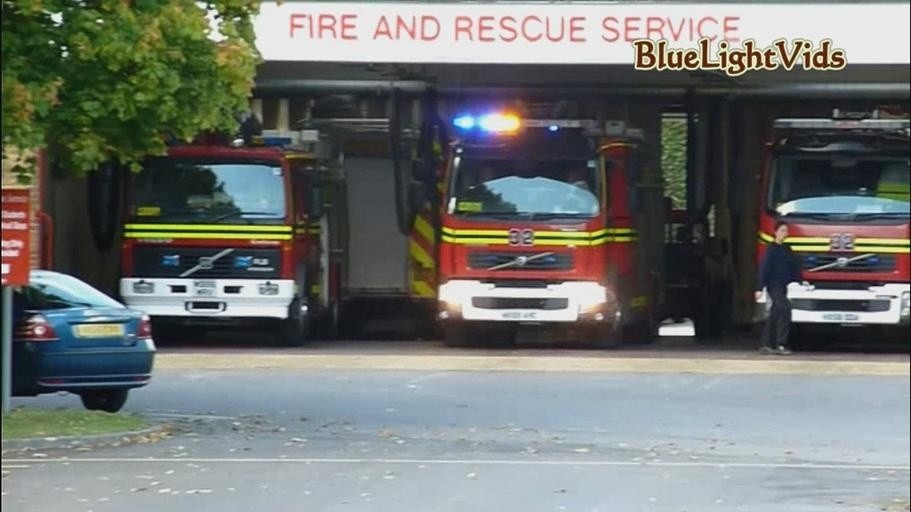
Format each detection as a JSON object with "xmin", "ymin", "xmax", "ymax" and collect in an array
[{"xmin": 12, "ymin": 270, "xmax": 156, "ymax": 412}]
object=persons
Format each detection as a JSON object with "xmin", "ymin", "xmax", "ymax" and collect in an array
[{"xmin": 754, "ymin": 220, "xmax": 817, "ymax": 354}]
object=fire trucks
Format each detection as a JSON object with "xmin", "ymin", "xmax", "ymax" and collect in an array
[
  {"xmin": 121, "ymin": 130, "xmax": 347, "ymax": 346},
  {"xmin": 406, "ymin": 112, "xmax": 665, "ymax": 349},
  {"xmin": 754, "ymin": 109, "xmax": 910, "ymax": 324}
]
